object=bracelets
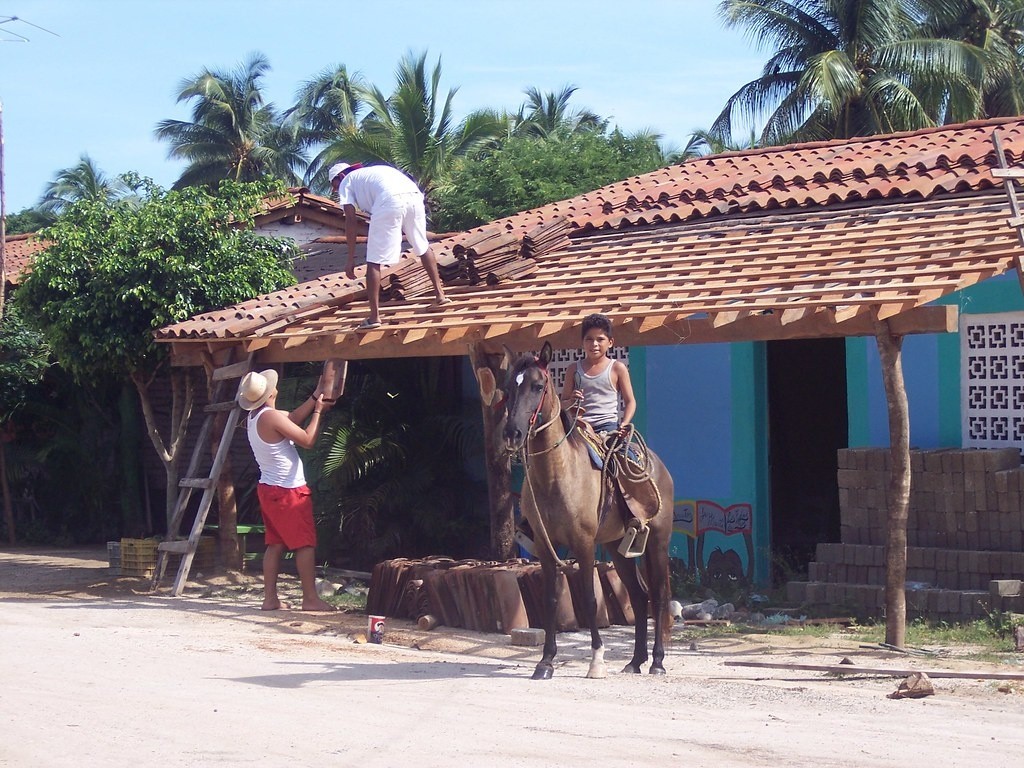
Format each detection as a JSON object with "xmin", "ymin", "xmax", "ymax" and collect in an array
[
  {"xmin": 311, "ymin": 394, "xmax": 317, "ymax": 401},
  {"xmin": 313, "ymin": 411, "xmax": 320, "ymax": 414}
]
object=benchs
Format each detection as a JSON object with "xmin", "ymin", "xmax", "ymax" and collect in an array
[{"xmin": 208, "ymin": 523, "xmax": 295, "ymax": 565}]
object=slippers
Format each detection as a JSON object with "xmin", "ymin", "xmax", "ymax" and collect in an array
[
  {"xmin": 358, "ymin": 319, "xmax": 381, "ymax": 329},
  {"xmin": 439, "ymin": 298, "xmax": 452, "ymax": 305}
]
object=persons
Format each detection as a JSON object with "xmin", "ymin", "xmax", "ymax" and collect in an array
[
  {"xmin": 328, "ymin": 162, "xmax": 451, "ymax": 328},
  {"xmin": 238, "ymin": 370, "xmax": 347, "ymax": 613},
  {"xmin": 515, "ymin": 314, "xmax": 637, "ymax": 544}
]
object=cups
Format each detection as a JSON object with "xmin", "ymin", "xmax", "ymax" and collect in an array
[{"xmin": 367, "ymin": 615, "xmax": 386, "ymax": 644}]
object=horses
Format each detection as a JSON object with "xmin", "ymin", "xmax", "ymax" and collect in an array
[{"xmin": 491, "ymin": 339, "xmax": 678, "ymax": 682}]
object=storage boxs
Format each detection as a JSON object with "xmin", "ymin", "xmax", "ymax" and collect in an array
[{"xmin": 107, "ymin": 536, "xmax": 216, "ymax": 577}]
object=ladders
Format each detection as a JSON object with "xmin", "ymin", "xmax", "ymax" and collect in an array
[{"xmin": 150, "ymin": 348, "xmax": 257, "ymax": 596}]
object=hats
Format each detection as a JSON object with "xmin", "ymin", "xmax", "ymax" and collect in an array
[
  {"xmin": 239, "ymin": 369, "xmax": 278, "ymax": 410},
  {"xmin": 328, "ymin": 163, "xmax": 363, "ymax": 183}
]
